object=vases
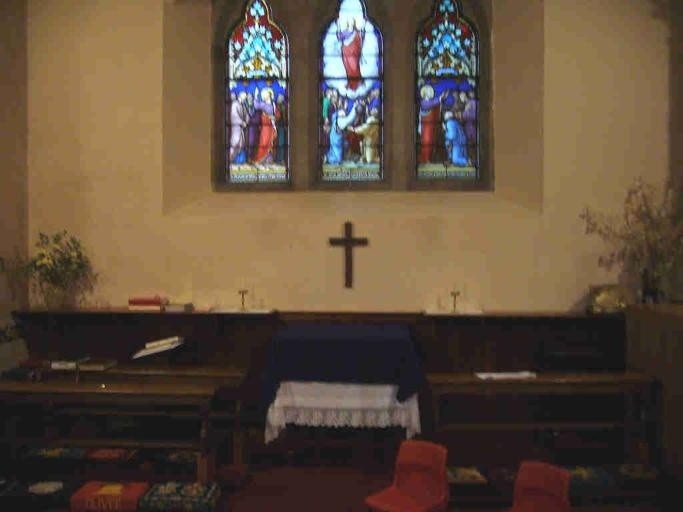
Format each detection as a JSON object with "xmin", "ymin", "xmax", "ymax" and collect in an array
[{"xmin": 43, "ymin": 282, "xmax": 68, "ymax": 310}]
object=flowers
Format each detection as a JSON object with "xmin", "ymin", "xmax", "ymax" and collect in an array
[{"xmin": 8, "ymin": 226, "xmax": 101, "ymax": 310}]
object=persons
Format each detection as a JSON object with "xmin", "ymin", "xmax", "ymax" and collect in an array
[{"xmin": 227, "ymin": 82, "xmax": 477, "ymax": 171}]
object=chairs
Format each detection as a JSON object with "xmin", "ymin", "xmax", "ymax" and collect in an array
[{"xmin": 364, "ymin": 440, "xmax": 449, "ymax": 512}]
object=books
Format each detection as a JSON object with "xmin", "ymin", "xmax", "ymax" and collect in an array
[{"xmin": 50, "ymin": 354, "xmax": 119, "ymax": 371}]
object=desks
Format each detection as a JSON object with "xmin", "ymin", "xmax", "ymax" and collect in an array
[
  {"xmin": 0, "ymin": 354, "xmax": 250, "ymax": 512},
  {"xmin": 257, "ymin": 321, "xmax": 422, "ymax": 470},
  {"xmin": 423, "ymin": 370, "xmax": 663, "ymax": 512}
]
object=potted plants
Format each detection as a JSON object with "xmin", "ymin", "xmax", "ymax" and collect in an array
[{"xmin": 579, "ymin": 174, "xmax": 683, "ymax": 312}]
